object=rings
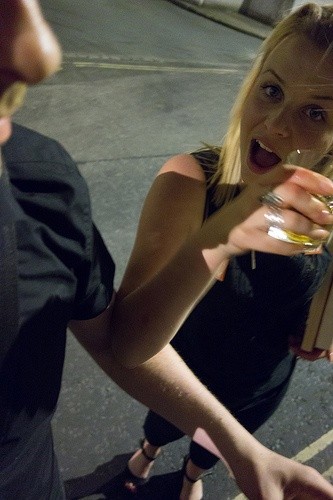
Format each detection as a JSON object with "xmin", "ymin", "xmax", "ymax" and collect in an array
[
  {"xmin": 264, "ymin": 203, "xmax": 286, "ymax": 229},
  {"xmin": 258, "ymin": 191, "xmax": 281, "ymax": 205}
]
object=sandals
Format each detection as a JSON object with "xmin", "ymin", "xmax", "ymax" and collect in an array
[
  {"xmin": 121, "ymin": 439, "xmax": 156, "ymax": 493},
  {"xmin": 178, "ymin": 454, "xmax": 214, "ymax": 500}
]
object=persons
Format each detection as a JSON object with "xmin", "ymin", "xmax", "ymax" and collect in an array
[
  {"xmin": 109, "ymin": 0, "xmax": 333, "ymax": 500},
  {"xmin": 0, "ymin": 0, "xmax": 333, "ymax": 500}
]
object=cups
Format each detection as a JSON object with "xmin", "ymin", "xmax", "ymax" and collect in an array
[{"xmin": 268, "ymin": 151, "xmax": 333, "ymax": 253}]
